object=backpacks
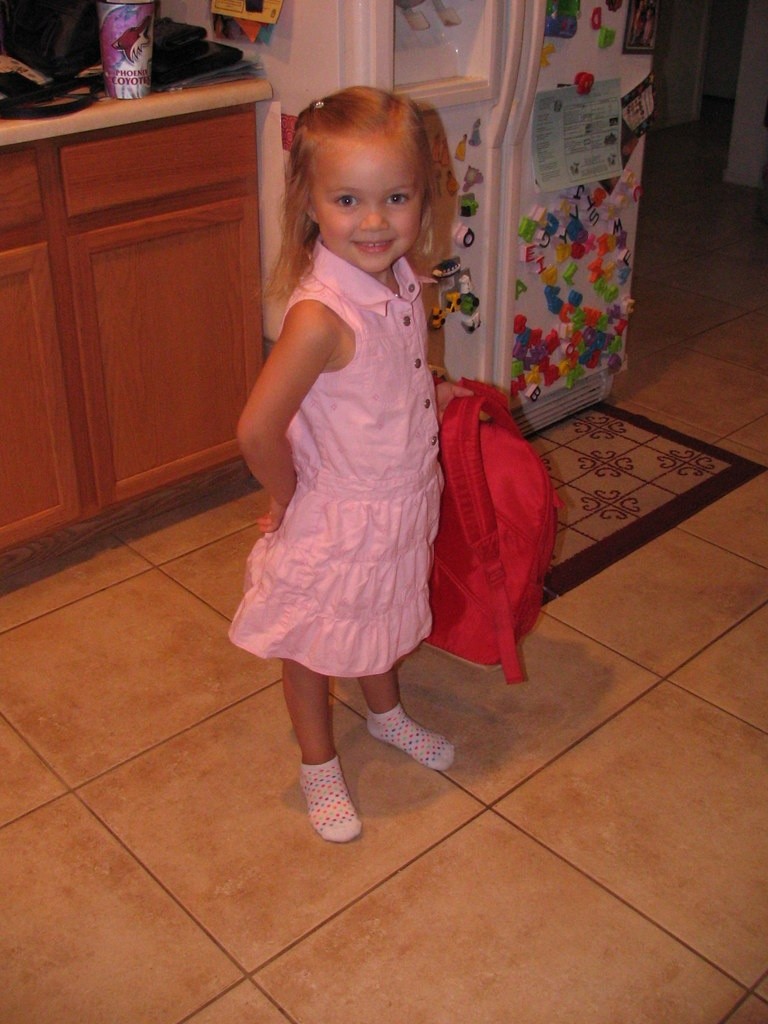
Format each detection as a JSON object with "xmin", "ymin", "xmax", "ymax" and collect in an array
[{"xmin": 416, "ymin": 374, "xmax": 560, "ymax": 685}]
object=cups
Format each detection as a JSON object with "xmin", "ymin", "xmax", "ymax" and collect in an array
[{"xmin": 96, "ymin": 0, "xmax": 158, "ymax": 100}]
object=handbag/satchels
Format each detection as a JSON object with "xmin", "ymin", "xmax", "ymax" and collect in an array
[
  {"xmin": 0, "ymin": 0, "xmax": 100, "ymax": 80},
  {"xmin": 146, "ymin": 17, "xmax": 243, "ymax": 84}
]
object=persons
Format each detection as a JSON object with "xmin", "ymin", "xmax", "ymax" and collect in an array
[{"xmin": 228, "ymin": 87, "xmax": 492, "ymax": 842}]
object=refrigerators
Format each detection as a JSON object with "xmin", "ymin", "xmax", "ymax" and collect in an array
[{"xmin": 160, "ymin": 0, "xmax": 659, "ymax": 438}]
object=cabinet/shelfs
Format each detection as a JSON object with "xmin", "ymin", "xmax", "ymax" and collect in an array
[{"xmin": 0, "ymin": 101, "xmax": 265, "ymax": 582}]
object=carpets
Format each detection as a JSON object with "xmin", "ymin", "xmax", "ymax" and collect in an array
[{"xmin": 522, "ymin": 402, "xmax": 768, "ymax": 610}]
